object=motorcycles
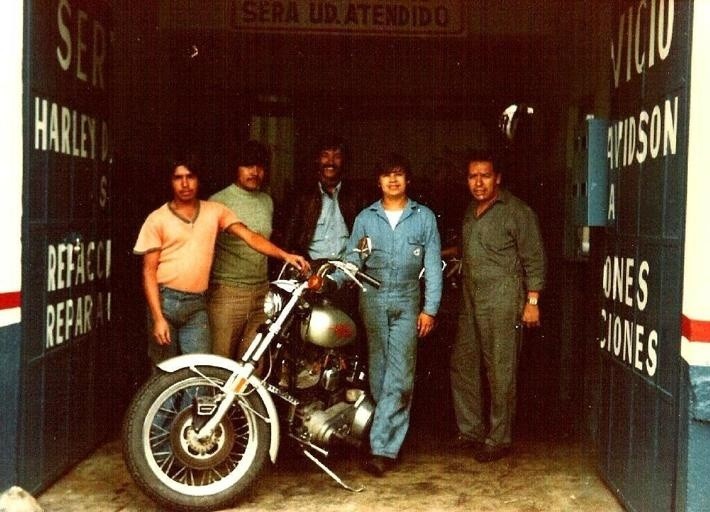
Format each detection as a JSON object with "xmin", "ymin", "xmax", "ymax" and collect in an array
[{"xmin": 119, "ymin": 257, "xmax": 465, "ymax": 512}]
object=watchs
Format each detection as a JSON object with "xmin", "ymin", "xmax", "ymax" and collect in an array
[{"xmin": 526, "ymin": 297, "xmax": 539, "ymax": 306}]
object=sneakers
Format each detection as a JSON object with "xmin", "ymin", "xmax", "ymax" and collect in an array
[
  {"xmin": 360, "ymin": 454, "xmax": 385, "ymax": 476},
  {"xmin": 447, "ymin": 425, "xmax": 512, "ymax": 462}
]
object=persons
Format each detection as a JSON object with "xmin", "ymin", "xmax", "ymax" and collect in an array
[
  {"xmin": 284, "ymin": 139, "xmax": 361, "ymax": 268},
  {"xmin": 206, "ymin": 140, "xmax": 275, "ymax": 375},
  {"xmin": 330, "ymin": 152, "xmax": 443, "ymax": 477},
  {"xmin": 132, "ymin": 152, "xmax": 311, "ymax": 463},
  {"xmin": 442, "ymin": 152, "xmax": 546, "ymax": 463}
]
